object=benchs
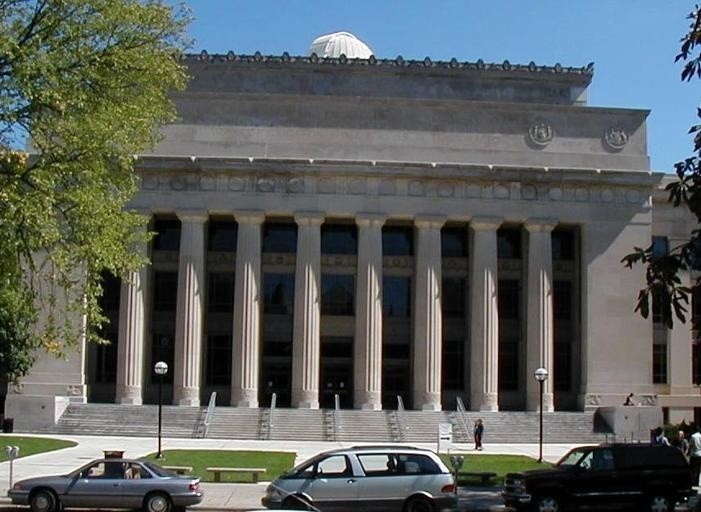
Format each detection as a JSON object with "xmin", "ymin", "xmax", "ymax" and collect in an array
[
  {"xmin": 206, "ymin": 468, "xmax": 266, "ymax": 482},
  {"xmin": 453, "ymin": 471, "xmax": 497, "ymax": 484},
  {"xmin": 161, "ymin": 466, "xmax": 193, "ymax": 475}
]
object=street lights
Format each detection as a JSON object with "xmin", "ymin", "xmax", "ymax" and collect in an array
[
  {"xmin": 153, "ymin": 360, "xmax": 169, "ymax": 462},
  {"xmin": 534, "ymin": 366, "xmax": 549, "ymax": 463}
]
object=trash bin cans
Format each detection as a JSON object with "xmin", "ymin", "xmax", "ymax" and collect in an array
[
  {"xmin": 103, "ymin": 450, "xmax": 125, "ymax": 474},
  {"xmin": 3, "ymin": 417, "xmax": 14, "ymax": 433}
]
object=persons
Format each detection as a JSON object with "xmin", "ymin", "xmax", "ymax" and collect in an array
[
  {"xmin": 475, "ymin": 419, "xmax": 485, "ymax": 450},
  {"xmin": 671, "ymin": 428, "xmax": 691, "ymax": 458},
  {"xmin": 651, "ymin": 427, "xmax": 675, "ymax": 447},
  {"xmin": 686, "ymin": 425, "xmax": 701, "ymax": 487},
  {"xmin": 473, "ymin": 419, "xmax": 481, "ymax": 451},
  {"xmin": 625, "ymin": 391, "xmax": 636, "ymax": 406},
  {"xmin": 385, "ymin": 460, "xmax": 396, "ymax": 472}
]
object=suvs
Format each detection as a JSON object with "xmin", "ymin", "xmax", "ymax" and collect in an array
[{"xmin": 498, "ymin": 441, "xmax": 697, "ymax": 512}]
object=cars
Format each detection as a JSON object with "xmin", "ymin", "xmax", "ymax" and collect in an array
[{"xmin": 7, "ymin": 458, "xmax": 206, "ymax": 511}]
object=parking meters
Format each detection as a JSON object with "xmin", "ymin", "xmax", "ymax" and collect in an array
[
  {"xmin": 5, "ymin": 445, "xmax": 19, "ymax": 490},
  {"xmin": 450, "ymin": 455, "xmax": 465, "ymax": 496}
]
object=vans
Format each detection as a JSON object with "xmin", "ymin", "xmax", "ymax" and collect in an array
[{"xmin": 258, "ymin": 445, "xmax": 458, "ymax": 511}]
